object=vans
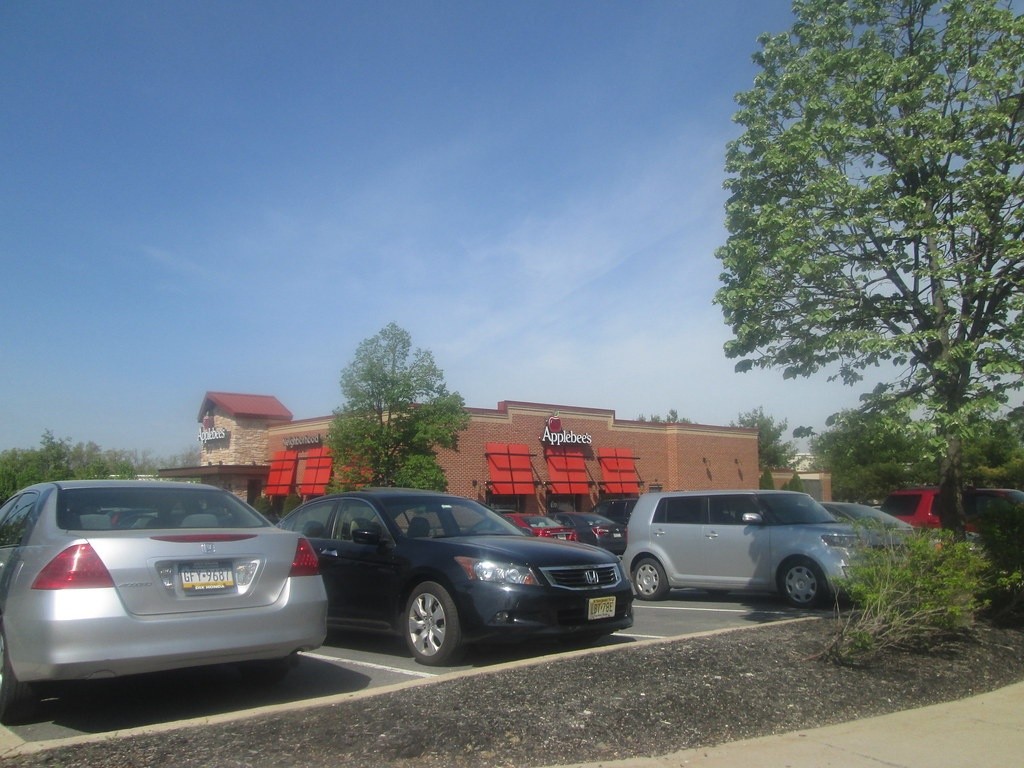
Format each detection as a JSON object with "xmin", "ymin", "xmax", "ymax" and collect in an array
[
  {"xmin": 623, "ymin": 490, "xmax": 873, "ymax": 609},
  {"xmin": 586, "ymin": 497, "xmax": 638, "ymax": 525}
]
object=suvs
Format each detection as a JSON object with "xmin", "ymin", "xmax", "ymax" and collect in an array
[{"xmin": 883, "ymin": 488, "xmax": 1024, "ymax": 540}]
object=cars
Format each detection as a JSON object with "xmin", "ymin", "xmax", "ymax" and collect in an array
[
  {"xmin": 272, "ymin": 487, "xmax": 635, "ymax": 666},
  {"xmin": 816, "ymin": 502, "xmax": 920, "ymax": 569},
  {"xmin": 489, "ymin": 508, "xmax": 578, "ymax": 542},
  {"xmin": 544, "ymin": 512, "xmax": 627, "ymax": 555},
  {"xmin": 0, "ymin": 479, "xmax": 328, "ymax": 724}
]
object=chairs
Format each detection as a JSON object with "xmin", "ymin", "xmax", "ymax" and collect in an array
[
  {"xmin": 350, "ymin": 517, "xmax": 370, "ymax": 538},
  {"xmin": 81, "ymin": 514, "xmax": 114, "ymax": 533},
  {"xmin": 183, "ymin": 513, "xmax": 218, "ymax": 527},
  {"xmin": 301, "ymin": 520, "xmax": 324, "ymax": 538}
]
